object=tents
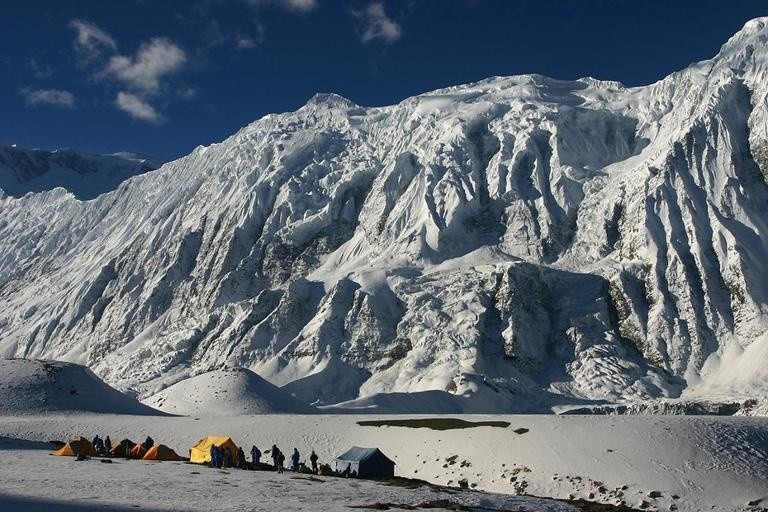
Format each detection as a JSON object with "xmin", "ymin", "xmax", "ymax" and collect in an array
[
  {"xmin": 335, "ymin": 446, "xmax": 396, "ymax": 480},
  {"xmin": 130, "ymin": 443, "xmax": 150, "ymax": 457},
  {"xmin": 189, "ymin": 434, "xmax": 239, "ymax": 465},
  {"xmin": 53, "ymin": 435, "xmax": 97, "ymax": 456},
  {"xmin": 143, "ymin": 444, "xmax": 184, "ymax": 461},
  {"xmin": 108, "ymin": 439, "xmax": 136, "ymax": 457}
]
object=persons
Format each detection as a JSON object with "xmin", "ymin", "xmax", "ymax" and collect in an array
[
  {"xmin": 146, "ymin": 436, "xmax": 154, "ymax": 448},
  {"xmin": 210, "ymin": 444, "xmax": 319, "ymax": 474},
  {"xmin": 125, "ymin": 439, "xmax": 131, "ymax": 456},
  {"xmin": 91, "ymin": 434, "xmax": 111, "ymax": 453}
]
